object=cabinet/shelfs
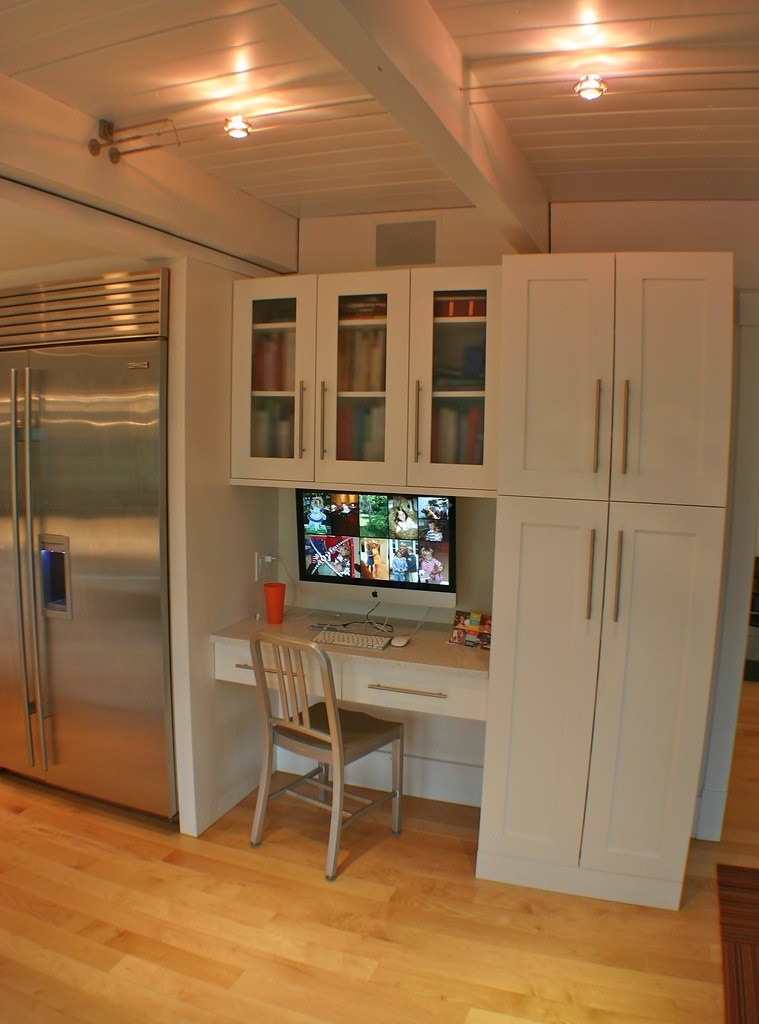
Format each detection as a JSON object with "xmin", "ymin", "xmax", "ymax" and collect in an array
[{"xmin": 231, "ymin": 251, "xmax": 740, "ymax": 912}]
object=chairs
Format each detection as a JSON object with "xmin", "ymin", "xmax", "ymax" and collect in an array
[{"xmin": 249, "ymin": 631, "xmax": 404, "ymax": 881}]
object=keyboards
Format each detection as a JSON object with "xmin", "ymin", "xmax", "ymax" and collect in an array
[{"xmin": 310, "ymin": 630, "xmax": 394, "ymax": 651}]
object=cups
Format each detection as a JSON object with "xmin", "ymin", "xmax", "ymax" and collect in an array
[{"xmin": 264, "ymin": 582, "xmax": 286, "ymax": 624}]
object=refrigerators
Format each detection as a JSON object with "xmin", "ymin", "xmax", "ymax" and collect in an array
[{"xmin": 0, "ymin": 338, "xmax": 179, "ymax": 822}]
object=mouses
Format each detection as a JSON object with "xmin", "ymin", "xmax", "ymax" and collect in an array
[{"xmin": 390, "ymin": 634, "xmax": 411, "ymax": 647}]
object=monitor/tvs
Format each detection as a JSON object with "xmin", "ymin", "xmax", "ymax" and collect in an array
[{"xmin": 295, "ymin": 489, "xmax": 458, "ymax": 594}]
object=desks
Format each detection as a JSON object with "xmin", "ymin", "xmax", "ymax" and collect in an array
[{"xmin": 210, "ymin": 605, "xmax": 489, "ymax": 723}]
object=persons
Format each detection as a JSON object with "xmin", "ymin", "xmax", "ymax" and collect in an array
[
  {"xmin": 420, "ymin": 547, "xmax": 444, "ymax": 583},
  {"xmin": 367, "ymin": 543, "xmax": 381, "ymax": 576},
  {"xmin": 456, "ymin": 615, "xmax": 469, "ymax": 627},
  {"xmin": 306, "ymin": 537, "xmax": 350, "ymax": 575},
  {"xmin": 392, "ymin": 542, "xmax": 418, "ymax": 583},
  {"xmin": 394, "ymin": 507, "xmax": 418, "ymax": 534},
  {"xmin": 425, "ymin": 499, "xmax": 448, "ymax": 541}
]
